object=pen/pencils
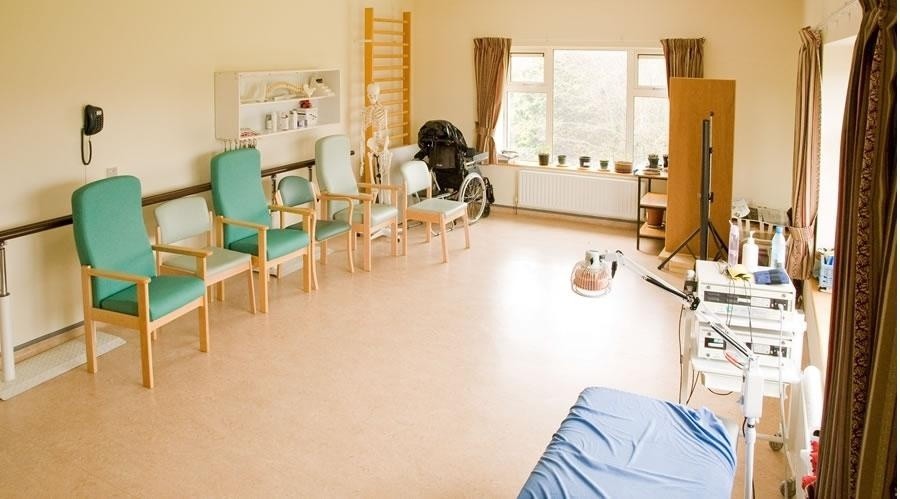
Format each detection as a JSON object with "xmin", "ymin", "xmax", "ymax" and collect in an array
[{"xmin": 821, "ymin": 249, "xmax": 834, "ymax": 265}]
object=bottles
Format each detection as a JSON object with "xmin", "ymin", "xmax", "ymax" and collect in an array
[
  {"xmin": 727, "ymin": 225, "xmax": 740, "ymax": 268},
  {"xmin": 265, "ymin": 110, "xmax": 305, "ymax": 132},
  {"xmin": 770, "ymin": 226, "xmax": 787, "ymax": 270}
]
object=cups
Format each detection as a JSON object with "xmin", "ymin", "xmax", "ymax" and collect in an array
[
  {"xmin": 646, "ymin": 207, "xmax": 664, "ymax": 226},
  {"xmin": 600, "ymin": 159, "xmax": 609, "ymax": 169},
  {"xmin": 648, "ymin": 155, "xmax": 659, "ymax": 167},
  {"xmin": 538, "ymin": 154, "xmax": 549, "ymax": 165},
  {"xmin": 819, "ymin": 262, "xmax": 833, "ymax": 294},
  {"xmin": 579, "ymin": 156, "xmax": 591, "ymax": 167},
  {"xmin": 558, "ymin": 155, "xmax": 566, "ymax": 164}
]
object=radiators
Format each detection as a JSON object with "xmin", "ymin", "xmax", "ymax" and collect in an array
[{"xmin": 796, "ymin": 365, "xmax": 823, "ymax": 499}]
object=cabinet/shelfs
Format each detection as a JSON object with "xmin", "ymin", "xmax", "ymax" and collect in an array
[
  {"xmin": 635, "ymin": 78, "xmax": 736, "ymax": 263},
  {"xmin": 214, "ymin": 66, "xmax": 345, "ymax": 140}
]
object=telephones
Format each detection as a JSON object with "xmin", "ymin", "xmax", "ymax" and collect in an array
[{"xmin": 84, "ymin": 105, "xmax": 103, "ymax": 135}]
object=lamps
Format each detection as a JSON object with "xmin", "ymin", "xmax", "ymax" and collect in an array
[{"xmin": 570, "ymin": 247, "xmax": 766, "ymax": 497}]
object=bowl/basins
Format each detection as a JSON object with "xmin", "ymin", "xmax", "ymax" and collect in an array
[{"xmin": 614, "ymin": 161, "xmax": 633, "ymax": 173}]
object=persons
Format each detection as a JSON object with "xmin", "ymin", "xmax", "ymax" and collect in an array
[{"xmin": 360, "ymin": 83, "xmax": 392, "ymax": 204}]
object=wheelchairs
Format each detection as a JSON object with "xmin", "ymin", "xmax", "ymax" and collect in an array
[{"xmin": 407, "ymin": 114, "xmax": 495, "ymax": 228}]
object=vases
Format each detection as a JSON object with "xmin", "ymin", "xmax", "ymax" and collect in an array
[{"xmin": 538, "ymin": 153, "xmax": 633, "ymax": 174}]
